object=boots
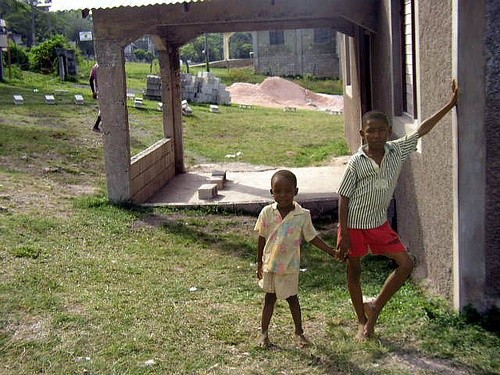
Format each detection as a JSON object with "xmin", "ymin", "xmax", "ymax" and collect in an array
[{"xmin": 92, "ymin": 115, "xmax": 101, "ymax": 132}]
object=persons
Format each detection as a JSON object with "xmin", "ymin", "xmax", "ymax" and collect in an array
[
  {"xmin": 254, "ymin": 170, "xmax": 345, "ymax": 350},
  {"xmin": 89, "ymin": 64, "xmax": 102, "ymax": 132},
  {"xmin": 337, "ymin": 78, "xmax": 459, "ymax": 343}
]
